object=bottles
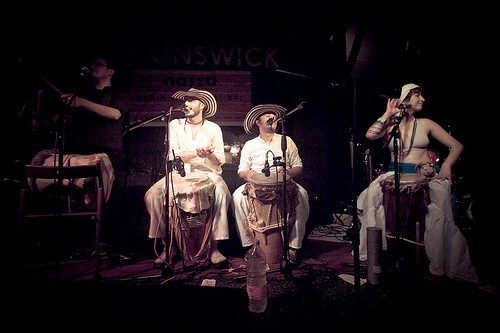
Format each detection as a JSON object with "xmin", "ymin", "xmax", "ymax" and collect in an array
[{"xmin": 247, "ymin": 239, "xmax": 267, "ymax": 312}]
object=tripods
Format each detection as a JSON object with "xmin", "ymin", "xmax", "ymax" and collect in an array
[
  {"xmin": 117, "ymin": 111, "xmax": 213, "ymax": 287},
  {"xmin": 236, "ymin": 101, "xmax": 318, "ymax": 294}
]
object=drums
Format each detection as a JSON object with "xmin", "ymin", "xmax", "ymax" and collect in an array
[
  {"xmin": 163, "ymin": 172, "xmax": 217, "ymax": 266},
  {"xmin": 26, "ymin": 148, "xmax": 115, "ymax": 210},
  {"xmin": 247, "ymin": 172, "xmax": 294, "ymax": 273},
  {"xmin": 380, "ymin": 173, "xmax": 430, "ymax": 274}
]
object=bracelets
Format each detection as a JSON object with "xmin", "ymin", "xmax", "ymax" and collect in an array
[{"xmin": 367, "ymin": 117, "xmax": 386, "ymax": 135}]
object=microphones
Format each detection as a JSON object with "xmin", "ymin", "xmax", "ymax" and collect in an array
[
  {"xmin": 264, "ymin": 152, "xmax": 270, "ymax": 177},
  {"xmin": 401, "ymin": 107, "xmax": 406, "ymax": 115},
  {"xmin": 267, "ymin": 118, "xmax": 273, "ymax": 125},
  {"xmin": 171, "ymin": 107, "xmax": 190, "ymax": 113},
  {"xmin": 80, "ymin": 67, "xmax": 88, "ymax": 80}
]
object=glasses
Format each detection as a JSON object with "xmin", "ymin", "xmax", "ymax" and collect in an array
[{"xmin": 93, "ymin": 62, "xmax": 108, "ymax": 68}]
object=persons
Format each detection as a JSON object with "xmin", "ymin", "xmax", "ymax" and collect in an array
[
  {"xmin": 143, "ymin": 87, "xmax": 231, "ymax": 266},
  {"xmin": 58, "ymin": 53, "xmax": 132, "ymax": 267},
  {"xmin": 351, "ymin": 82, "xmax": 480, "ymax": 286},
  {"xmin": 229, "ymin": 103, "xmax": 310, "ymax": 265}
]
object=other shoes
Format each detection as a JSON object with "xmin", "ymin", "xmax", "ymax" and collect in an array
[
  {"xmin": 154, "ymin": 252, "xmax": 168, "ymax": 266},
  {"xmin": 428, "ymin": 260, "xmax": 445, "ymax": 275},
  {"xmin": 286, "ymin": 249, "xmax": 297, "ymax": 263},
  {"xmin": 210, "ymin": 250, "xmax": 227, "ymax": 265}
]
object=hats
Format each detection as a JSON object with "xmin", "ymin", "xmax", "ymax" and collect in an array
[
  {"xmin": 243, "ymin": 104, "xmax": 287, "ymax": 135},
  {"xmin": 172, "ymin": 88, "xmax": 218, "ymax": 118}
]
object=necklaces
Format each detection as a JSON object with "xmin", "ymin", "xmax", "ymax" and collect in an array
[
  {"xmin": 186, "ymin": 119, "xmax": 204, "ymax": 125},
  {"xmin": 398, "ymin": 119, "xmax": 417, "ymax": 158}
]
